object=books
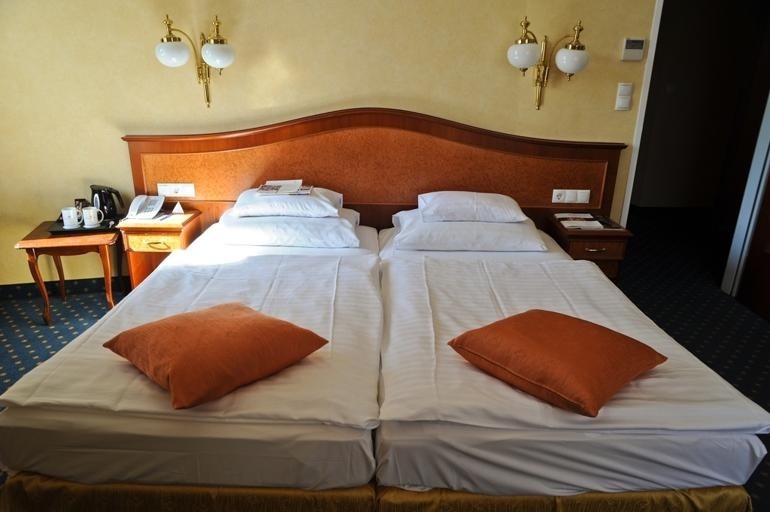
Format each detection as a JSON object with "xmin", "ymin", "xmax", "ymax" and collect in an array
[
  {"xmin": 554, "ymin": 212, "xmax": 623, "ymax": 230},
  {"xmin": 257, "ymin": 179, "xmax": 313, "ymax": 194}
]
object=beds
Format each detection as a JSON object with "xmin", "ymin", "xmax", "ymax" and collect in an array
[
  {"xmin": 0, "ymin": 212, "xmax": 379, "ymax": 510},
  {"xmin": 376, "ymin": 224, "xmax": 770, "ymax": 510}
]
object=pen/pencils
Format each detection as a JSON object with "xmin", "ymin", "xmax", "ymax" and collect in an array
[{"xmin": 160, "ymin": 215, "xmax": 171, "ymax": 221}]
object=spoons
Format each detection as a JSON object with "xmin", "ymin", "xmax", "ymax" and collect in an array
[{"xmin": 108, "ymin": 220, "xmax": 114, "ymax": 228}]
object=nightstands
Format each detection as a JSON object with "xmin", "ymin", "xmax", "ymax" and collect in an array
[
  {"xmin": 550, "ymin": 210, "xmax": 633, "ymax": 286},
  {"xmin": 117, "ymin": 205, "xmax": 203, "ymax": 292}
]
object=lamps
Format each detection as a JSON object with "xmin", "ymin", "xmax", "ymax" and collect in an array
[
  {"xmin": 157, "ymin": 12, "xmax": 236, "ymax": 111},
  {"xmin": 506, "ymin": 11, "xmax": 587, "ymax": 111}
]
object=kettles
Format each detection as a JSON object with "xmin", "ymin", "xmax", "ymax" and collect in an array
[{"xmin": 88, "ymin": 184, "xmax": 125, "ymax": 220}]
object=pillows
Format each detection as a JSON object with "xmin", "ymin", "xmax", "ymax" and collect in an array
[
  {"xmin": 388, "ymin": 208, "xmax": 548, "ymax": 253},
  {"xmin": 97, "ymin": 301, "xmax": 336, "ymax": 412},
  {"xmin": 415, "ymin": 191, "xmax": 530, "ymax": 224},
  {"xmin": 444, "ymin": 304, "xmax": 675, "ymax": 422},
  {"xmin": 238, "ymin": 186, "xmax": 341, "ymax": 216},
  {"xmin": 211, "ymin": 208, "xmax": 362, "ymax": 250}
]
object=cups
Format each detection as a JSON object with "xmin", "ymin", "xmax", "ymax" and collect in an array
[
  {"xmin": 61, "ymin": 205, "xmax": 83, "ymax": 229},
  {"xmin": 81, "ymin": 206, "xmax": 105, "ymax": 229}
]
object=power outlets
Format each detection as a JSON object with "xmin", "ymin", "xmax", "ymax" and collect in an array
[{"xmin": 550, "ymin": 189, "xmax": 566, "ymax": 203}]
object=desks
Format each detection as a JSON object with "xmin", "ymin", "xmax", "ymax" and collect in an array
[{"xmin": 13, "ymin": 213, "xmax": 120, "ymax": 325}]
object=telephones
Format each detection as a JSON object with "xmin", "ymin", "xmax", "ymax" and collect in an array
[{"xmin": 127, "ymin": 195, "xmax": 165, "ymax": 220}]
